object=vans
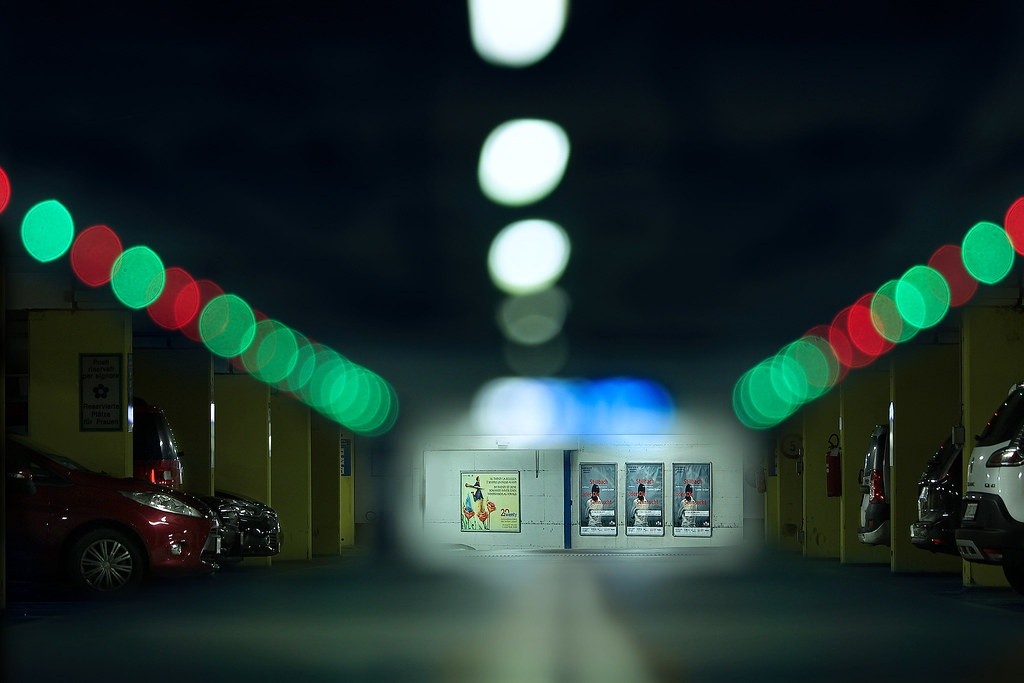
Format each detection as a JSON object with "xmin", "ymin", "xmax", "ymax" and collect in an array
[
  {"xmin": 128, "ymin": 393, "xmax": 188, "ymax": 495},
  {"xmin": 856, "ymin": 421, "xmax": 894, "ymax": 551}
]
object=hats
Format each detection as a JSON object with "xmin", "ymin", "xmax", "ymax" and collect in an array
[
  {"xmin": 685, "ymin": 483, "xmax": 693, "ymax": 493},
  {"xmin": 638, "ymin": 483, "xmax": 645, "ymax": 493},
  {"xmin": 592, "ymin": 484, "xmax": 599, "ymax": 492}
]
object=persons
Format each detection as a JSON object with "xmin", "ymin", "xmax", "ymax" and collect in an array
[
  {"xmin": 631, "ymin": 483, "xmax": 649, "ymax": 527},
  {"xmin": 584, "ymin": 483, "xmax": 603, "ymax": 527},
  {"xmin": 471, "ymin": 475, "xmax": 484, "ymax": 512},
  {"xmin": 677, "ymin": 483, "xmax": 698, "ymax": 527}
]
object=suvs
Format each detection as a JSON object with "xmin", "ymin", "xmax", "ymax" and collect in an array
[
  {"xmin": 960, "ymin": 380, "xmax": 1024, "ymax": 595},
  {"xmin": 908, "ymin": 423, "xmax": 960, "ymax": 556}
]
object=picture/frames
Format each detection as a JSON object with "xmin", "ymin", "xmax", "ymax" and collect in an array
[
  {"xmin": 671, "ymin": 462, "xmax": 712, "ymax": 539},
  {"xmin": 460, "ymin": 469, "xmax": 522, "ymax": 533},
  {"xmin": 578, "ymin": 461, "xmax": 618, "ymax": 537},
  {"xmin": 624, "ymin": 461, "xmax": 666, "ymax": 537}
]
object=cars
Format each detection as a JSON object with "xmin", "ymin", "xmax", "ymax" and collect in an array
[
  {"xmin": 0, "ymin": 424, "xmax": 221, "ymax": 605},
  {"xmin": 156, "ymin": 485, "xmax": 242, "ymax": 582},
  {"xmin": 224, "ymin": 477, "xmax": 282, "ymax": 558}
]
